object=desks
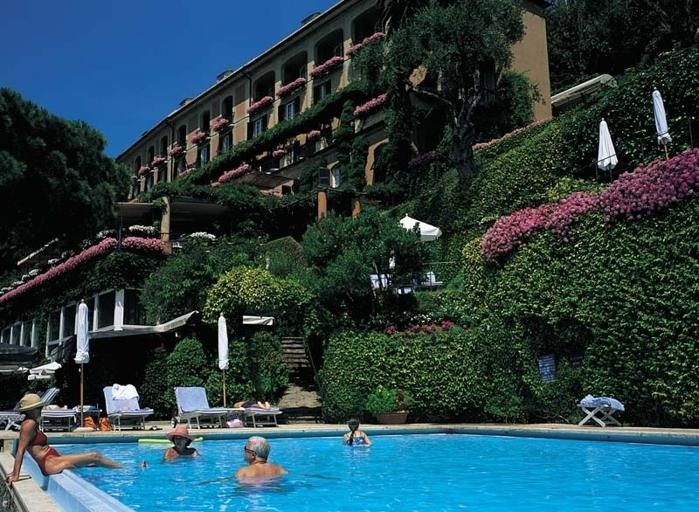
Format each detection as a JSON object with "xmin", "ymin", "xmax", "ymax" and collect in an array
[
  {"xmin": 576, "ymin": 404, "xmax": 622, "ymax": 427},
  {"xmin": 40, "ymin": 402, "xmax": 76, "ymax": 433},
  {"xmin": 76, "ymin": 403, "xmax": 102, "ymax": 432}
]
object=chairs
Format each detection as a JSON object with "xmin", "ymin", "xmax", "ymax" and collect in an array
[
  {"xmin": 0, "ymin": 388, "xmax": 60, "ymax": 430},
  {"xmin": 102, "ymin": 386, "xmax": 155, "ymax": 432},
  {"xmin": 171, "ymin": 386, "xmax": 229, "ymax": 429},
  {"xmin": 216, "ymin": 407, "xmax": 284, "ymax": 428}
]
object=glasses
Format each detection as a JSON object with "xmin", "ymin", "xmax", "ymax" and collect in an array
[{"xmin": 245, "ymin": 446, "xmax": 258, "ymax": 455}]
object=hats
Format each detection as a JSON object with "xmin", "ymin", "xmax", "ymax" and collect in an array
[
  {"xmin": 19, "ymin": 393, "xmax": 44, "ymax": 411},
  {"xmin": 167, "ymin": 427, "xmax": 193, "ymax": 446}
]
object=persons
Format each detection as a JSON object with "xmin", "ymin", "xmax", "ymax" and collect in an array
[
  {"xmin": 4, "ymin": 392, "xmax": 123, "ymax": 488},
  {"xmin": 233, "ymin": 435, "xmax": 288, "ymax": 485},
  {"xmin": 160, "ymin": 425, "xmax": 200, "ymax": 461},
  {"xmin": 233, "ymin": 399, "xmax": 270, "ymax": 410},
  {"xmin": 343, "ymin": 416, "xmax": 372, "ymax": 447}
]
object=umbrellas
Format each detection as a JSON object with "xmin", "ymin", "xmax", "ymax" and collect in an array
[
  {"xmin": 651, "ymin": 86, "xmax": 674, "ymax": 161},
  {"xmin": 73, "ymin": 298, "xmax": 91, "ymax": 427},
  {"xmin": 596, "ymin": 118, "xmax": 619, "ymax": 181},
  {"xmin": 217, "ymin": 311, "xmax": 230, "ymax": 407},
  {"xmin": 399, "ymin": 212, "xmax": 444, "ymax": 244}
]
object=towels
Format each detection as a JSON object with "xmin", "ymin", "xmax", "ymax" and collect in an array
[
  {"xmin": 580, "ymin": 394, "xmax": 626, "ymax": 412},
  {"xmin": 112, "ymin": 382, "xmax": 140, "ymax": 400}
]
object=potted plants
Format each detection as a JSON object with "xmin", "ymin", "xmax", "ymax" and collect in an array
[{"xmin": 362, "ymin": 383, "xmax": 416, "ymax": 424}]
